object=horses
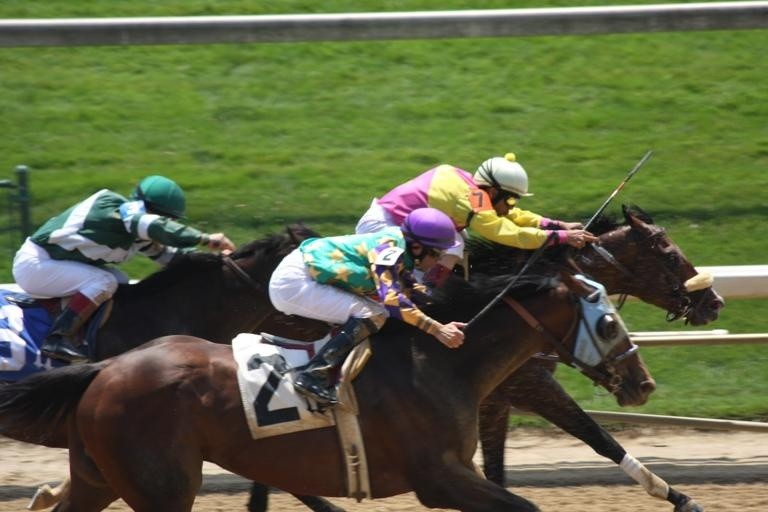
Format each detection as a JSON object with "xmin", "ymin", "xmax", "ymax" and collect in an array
[
  {"xmin": 1, "ymin": 247, "xmax": 657, "ymax": 512},
  {"xmin": 245, "ymin": 200, "xmax": 726, "ymax": 512},
  {"xmin": 0, "ymin": 222, "xmax": 325, "ymax": 362}
]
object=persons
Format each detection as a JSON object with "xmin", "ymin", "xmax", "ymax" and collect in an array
[
  {"xmin": 11, "ymin": 176, "xmax": 237, "ymax": 365},
  {"xmin": 355, "ymin": 152, "xmax": 602, "ymax": 290},
  {"xmin": 267, "ymin": 206, "xmax": 469, "ymax": 411}
]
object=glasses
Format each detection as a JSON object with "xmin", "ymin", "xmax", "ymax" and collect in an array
[
  {"xmin": 505, "ymin": 198, "xmax": 517, "ymax": 207},
  {"xmin": 426, "ymin": 250, "xmax": 446, "ymax": 259}
]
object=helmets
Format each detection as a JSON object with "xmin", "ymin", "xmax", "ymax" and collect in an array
[
  {"xmin": 132, "ymin": 175, "xmax": 186, "ymax": 218},
  {"xmin": 401, "ymin": 207, "xmax": 461, "ymax": 248},
  {"xmin": 472, "ymin": 157, "xmax": 534, "ymax": 197}
]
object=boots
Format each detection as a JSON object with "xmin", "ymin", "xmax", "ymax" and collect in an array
[
  {"xmin": 294, "ymin": 318, "xmax": 379, "ymax": 408},
  {"xmin": 40, "ymin": 292, "xmax": 98, "ymax": 363}
]
